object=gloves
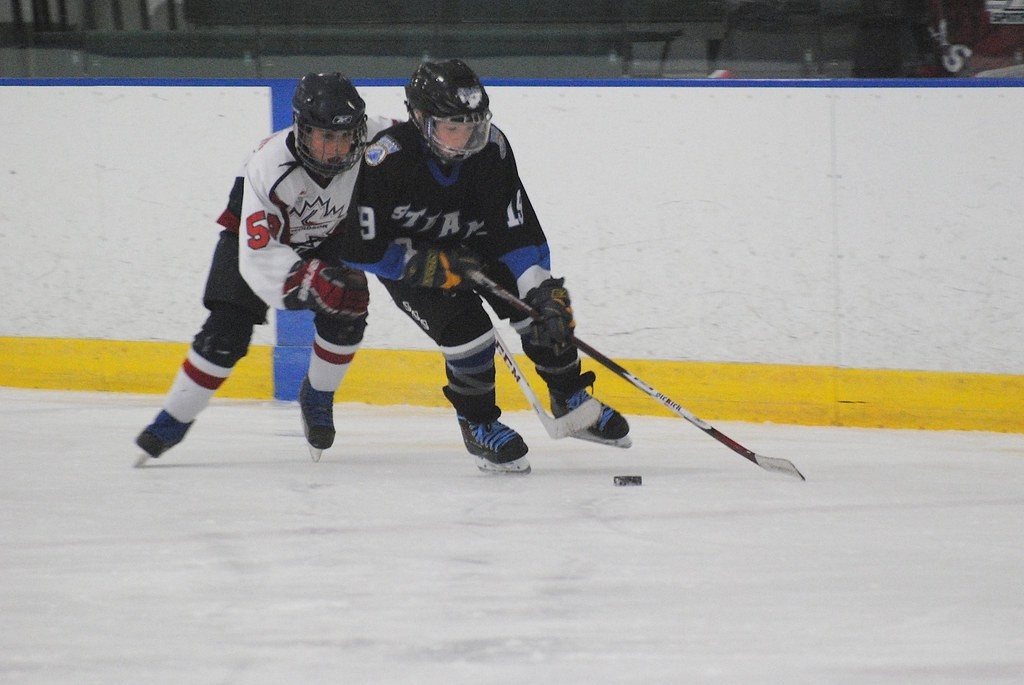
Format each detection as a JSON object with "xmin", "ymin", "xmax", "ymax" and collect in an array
[
  {"xmin": 282, "ymin": 258, "xmax": 370, "ymax": 323},
  {"xmin": 518, "ymin": 277, "xmax": 575, "ymax": 355},
  {"xmin": 404, "ymin": 243, "xmax": 491, "ymax": 291}
]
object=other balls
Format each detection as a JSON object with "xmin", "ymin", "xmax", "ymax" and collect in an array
[{"xmin": 613, "ymin": 474, "xmax": 643, "ymax": 485}]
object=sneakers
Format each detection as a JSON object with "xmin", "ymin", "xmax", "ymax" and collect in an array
[
  {"xmin": 133, "ymin": 408, "xmax": 195, "ymax": 467},
  {"xmin": 299, "ymin": 375, "xmax": 337, "ymax": 461},
  {"xmin": 534, "ymin": 368, "xmax": 632, "ymax": 448},
  {"xmin": 443, "ymin": 386, "xmax": 528, "ymax": 472}
]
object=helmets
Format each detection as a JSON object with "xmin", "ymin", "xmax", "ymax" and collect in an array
[
  {"xmin": 292, "ymin": 71, "xmax": 367, "ymax": 146},
  {"xmin": 405, "ymin": 58, "xmax": 491, "ymax": 136}
]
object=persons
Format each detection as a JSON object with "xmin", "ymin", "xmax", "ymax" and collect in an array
[
  {"xmin": 131, "ymin": 72, "xmax": 402, "ymax": 469},
  {"xmin": 709, "ymin": 1, "xmax": 1024, "ymax": 80},
  {"xmin": 340, "ymin": 59, "xmax": 632, "ymax": 474}
]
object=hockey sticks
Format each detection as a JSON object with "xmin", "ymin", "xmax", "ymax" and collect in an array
[
  {"xmin": 469, "ymin": 268, "xmax": 807, "ymax": 481},
  {"xmin": 476, "ymin": 294, "xmax": 604, "ymax": 440}
]
